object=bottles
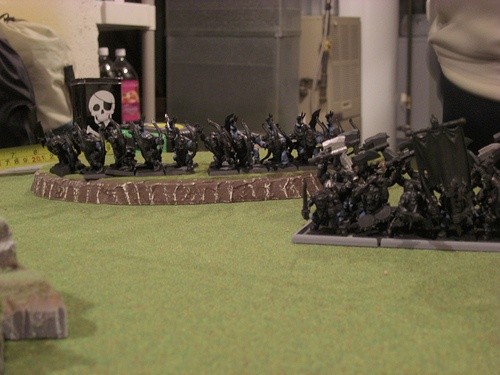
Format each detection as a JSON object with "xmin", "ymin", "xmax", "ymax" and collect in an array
[
  {"xmin": 97, "ymin": 47, "xmax": 119, "ymax": 79},
  {"xmin": 112, "ymin": 48, "xmax": 141, "ymax": 127}
]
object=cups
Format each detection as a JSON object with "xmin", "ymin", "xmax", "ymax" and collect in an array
[{"xmin": 68, "ymin": 78, "xmax": 123, "ymax": 134}]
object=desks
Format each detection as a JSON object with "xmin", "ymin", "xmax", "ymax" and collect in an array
[{"xmin": 0, "ymin": 119, "xmax": 500, "ymax": 374}]
